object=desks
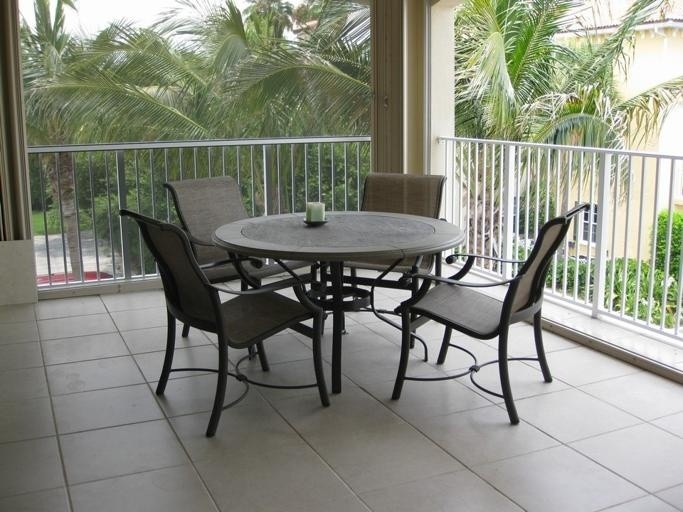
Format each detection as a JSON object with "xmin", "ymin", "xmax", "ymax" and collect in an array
[{"xmin": 215, "ymin": 211, "xmax": 464, "ymax": 394}]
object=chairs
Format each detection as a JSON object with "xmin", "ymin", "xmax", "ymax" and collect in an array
[
  {"xmin": 309, "ymin": 173, "xmax": 449, "ymax": 338},
  {"xmin": 119, "ymin": 204, "xmax": 330, "ymax": 435},
  {"xmin": 389, "ymin": 201, "xmax": 591, "ymax": 427},
  {"xmin": 163, "ymin": 175, "xmax": 317, "ymax": 364}
]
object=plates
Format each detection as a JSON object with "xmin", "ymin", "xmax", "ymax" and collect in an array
[{"xmin": 303, "ymin": 218, "xmax": 328, "ymax": 225}]
